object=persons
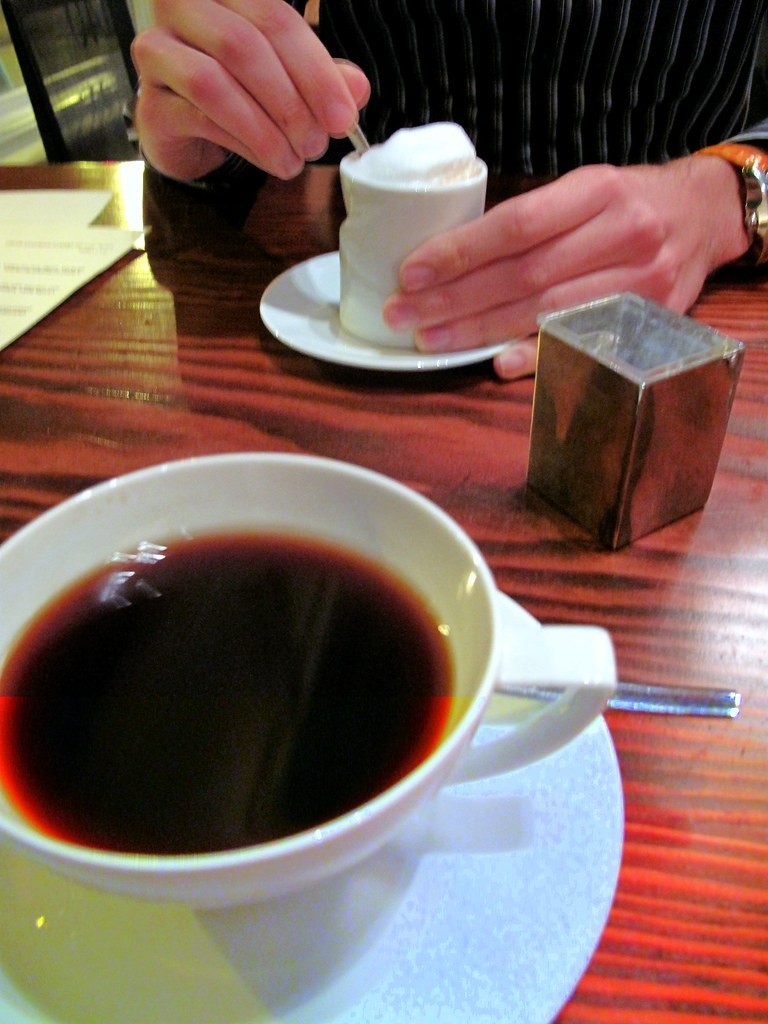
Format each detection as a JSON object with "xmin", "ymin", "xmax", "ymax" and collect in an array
[{"xmin": 125, "ymin": 0, "xmax": 768, "ymax": 381}]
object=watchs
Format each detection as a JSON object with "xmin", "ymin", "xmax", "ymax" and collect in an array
[{"xmin": 698, "ymin": 143, "xmax": 768, "ymax": 268}]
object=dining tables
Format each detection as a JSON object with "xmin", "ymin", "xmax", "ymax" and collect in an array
[{"xmin": 0, "ymin": 160, "xmax": 768, "ymax": 1024}]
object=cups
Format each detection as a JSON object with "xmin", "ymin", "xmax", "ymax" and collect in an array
[
  {"xmin": 0, "ymin": 449, "xmax": 618, "ymax": 905},
  {"xmin": 339, "ymin": 144, "xmax": 488, "ymax": 349}
]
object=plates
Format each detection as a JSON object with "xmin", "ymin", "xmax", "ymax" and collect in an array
[
  {"xmin": 258, "ymin": 249, "xmax": 516, "ymax": 371},
  {"xmin": 0, "ymin": 590, "xmax": 626, "ymax": 1023}
]
